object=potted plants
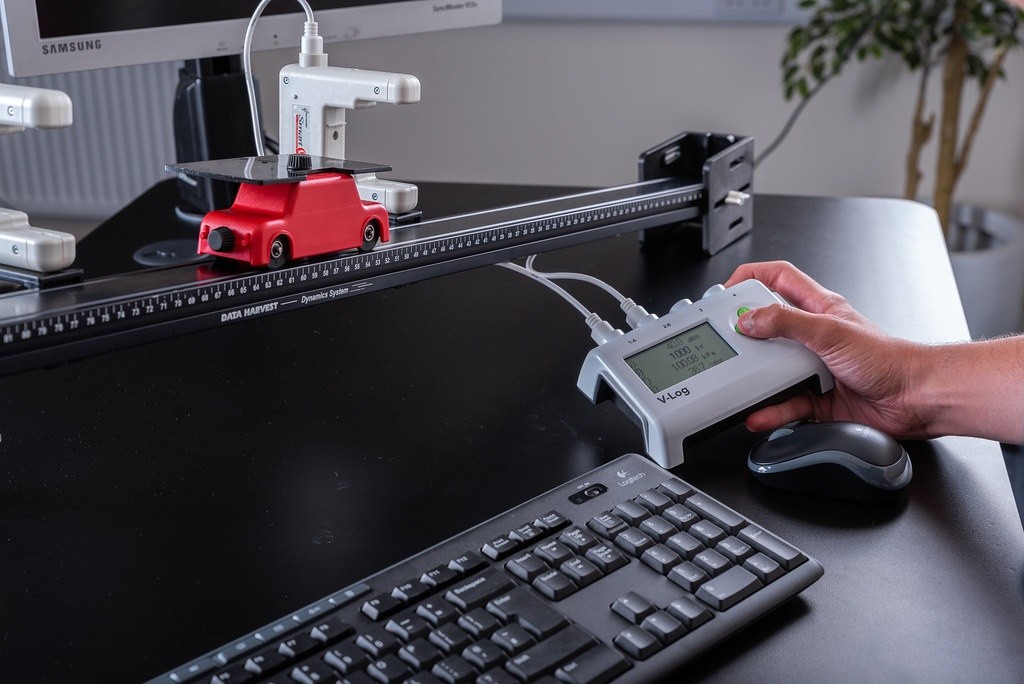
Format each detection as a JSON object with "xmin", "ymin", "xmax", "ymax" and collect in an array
[{"xmin": 779, "ymin": 0, "xmax": 1024, "ymax": 337}]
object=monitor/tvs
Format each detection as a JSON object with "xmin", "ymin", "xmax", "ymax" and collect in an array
[{"xmin": 0, "ymin": 0, "xmax": 503, "ymax": 80}]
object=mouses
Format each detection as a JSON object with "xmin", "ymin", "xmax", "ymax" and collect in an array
[{"xmin": 747, "ymin": 421, "xmax": 912, "ymax": 492}]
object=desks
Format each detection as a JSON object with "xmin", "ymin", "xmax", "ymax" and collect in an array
[{"xmin": 0, "ymin": 180, "xmax": 1024, "ymax": 684}]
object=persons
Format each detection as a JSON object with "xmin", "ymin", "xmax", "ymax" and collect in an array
[{"xmin": 723, "ymin": 260, "xmax": 1024, "ymax": 447}]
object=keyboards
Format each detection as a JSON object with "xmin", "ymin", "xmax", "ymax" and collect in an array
[{"xmin": 136, "ymin": 453, "xmax": 827, "ymax": 684}]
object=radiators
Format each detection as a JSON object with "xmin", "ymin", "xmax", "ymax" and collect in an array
[{"xmin": 0, "ymin": 60, "xmax": 187, "ymax": 220}]
